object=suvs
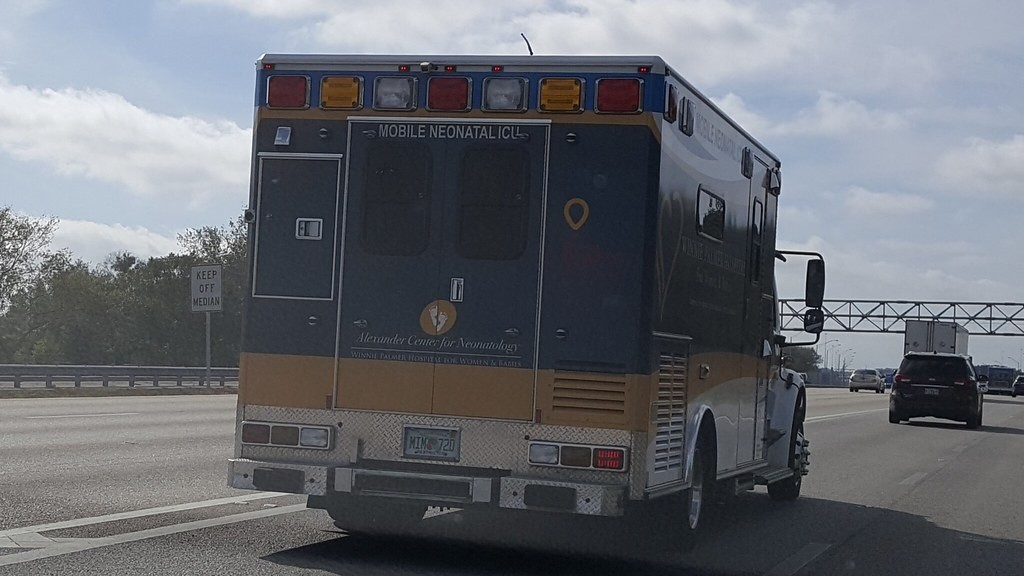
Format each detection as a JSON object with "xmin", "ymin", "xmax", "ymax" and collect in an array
[
  {"xmin": 888, "ymin": 349, "xmax": 990, "ymax": 430},
  {"xmin": 1011, "ymin": 374, "xmax": 1024, "ymax": 397}
]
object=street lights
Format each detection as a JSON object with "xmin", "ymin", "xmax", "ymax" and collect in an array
[
  {"xmin": 825, "ymin": 339, "xmax": 839, "ymax": 369},
  {"xmin": 831, "ymin": 344, "xmax": 842, "ymax": 385},
  {"xmin": 1007, "ymin": 356, "xmax": 1020, "ymax": 374},
  {"xmin": 843, "ymin": 352, "xmax": 857, "ymax": 383}
]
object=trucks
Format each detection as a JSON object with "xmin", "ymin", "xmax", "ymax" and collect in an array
[{"xmin": 902, "ymin": 320, "xmax": 970, "ymax": 356}]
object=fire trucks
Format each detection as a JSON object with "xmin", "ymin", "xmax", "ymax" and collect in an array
[{"xmin": 227, "ymin": 52, "xmax": 828, "ymax": 541}]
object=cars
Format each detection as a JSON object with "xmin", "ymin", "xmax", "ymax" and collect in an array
[{"xmin": 848, "ymin": 367, "xmax": 887, "ymax": 394}]
